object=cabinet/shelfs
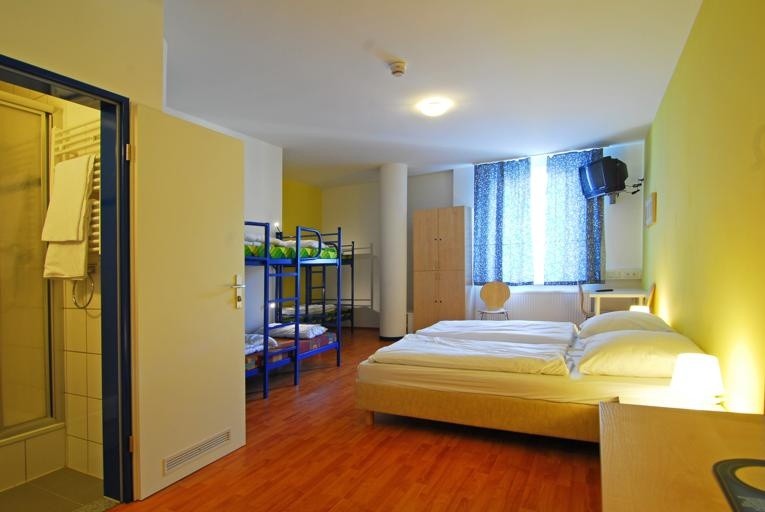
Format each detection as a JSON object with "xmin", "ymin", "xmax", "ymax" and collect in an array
[{"xmin": 412, "ymin": 206, "xmax": 473, "ymax": 333}]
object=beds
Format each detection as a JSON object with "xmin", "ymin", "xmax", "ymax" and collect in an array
[
  {"xmin": 244, "ymin": 221, "xmax": 355, "ymax": 399},
  {"xmin": 354, "ymin": 320, "xmax": 710, "ymax": 443}
]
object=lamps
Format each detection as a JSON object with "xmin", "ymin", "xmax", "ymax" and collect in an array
[
  {"xmin": 629, "ymin": 305, "xmax": 650, "ymax": 314},
  {"xmin": 415, "ymin": 96, "xmax": 453, "ymax": 117},
  {"xmin": 670, "ymin": 353, "xmax": 724, "ymax": 412}
]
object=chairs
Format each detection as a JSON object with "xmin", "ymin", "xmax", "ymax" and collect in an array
[
  {"xmin": 478, "ymin": 282, "xmax": 511, "ymax": 320},
  {"xmin": 577, "ymin": 282, "xmax": 595, "ymax": 322}
]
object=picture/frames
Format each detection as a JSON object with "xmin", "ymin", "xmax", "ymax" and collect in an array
[{"xmin": 644, "ymin": 192, "xmax": 657, "ymax": 227}]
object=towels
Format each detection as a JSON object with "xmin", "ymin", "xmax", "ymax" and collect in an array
[
  {"xmin": 43, "ymin": 200, "xmax": 93, "ymax": 281},
  {"xmin": 41, "ymin": 153, "xmax": 96, "ymax": 242}
]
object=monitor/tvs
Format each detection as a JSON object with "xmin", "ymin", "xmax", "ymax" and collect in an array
[{"xmin": 578, "ymin": 155, "xmax": 628, "ymax": 198}]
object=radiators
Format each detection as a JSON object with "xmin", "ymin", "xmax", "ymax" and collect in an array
[
  {"xmin": 487, "ymin": 292, "xmax": 590, "ymax": 324},
  {"xmin": 49, "ymin": 118, "xmax": 102, "ymax": 256}
]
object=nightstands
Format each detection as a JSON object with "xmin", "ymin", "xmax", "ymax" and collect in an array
[{"xmin": 618, "ymin": 396, "xmax": 726, "ymax": 412}]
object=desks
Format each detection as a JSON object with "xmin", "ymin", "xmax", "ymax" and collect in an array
[
  {"xmin": 589, "ymin": 288, "xmax": 645, "ymax": 317},
  {"xmin": 599, "ymin": 401, "xmax": 765, "ymax": 512}
]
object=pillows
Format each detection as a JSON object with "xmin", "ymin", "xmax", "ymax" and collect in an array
[
  {"xmin": 578, "ymin": 311, "xmax": 674, "ymax": 338},
  {"xmin": 580, "ymin": 330, "xmax": 706, "ymax": 378}
]
